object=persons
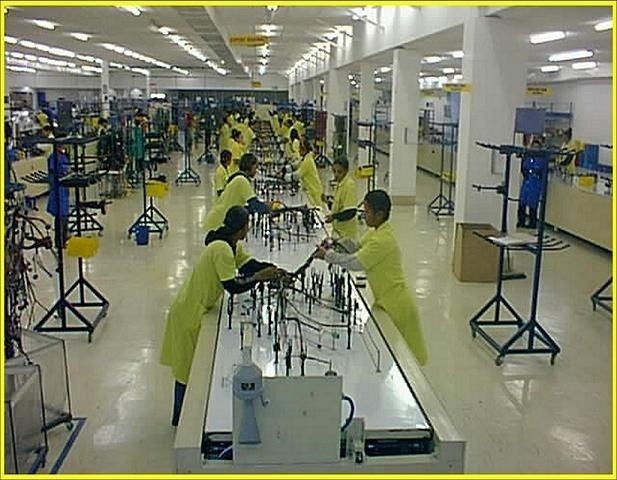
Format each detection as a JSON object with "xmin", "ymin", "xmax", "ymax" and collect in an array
[
  {"xmin": 556, "ymin": 128, "xmax": 577, "ymax": 177},
  {"xmin": 517, "ymin": 140, "xmax": 548, "ymax": 229},
  {"xmin": 29, "ymin": 101, "xmax": 149, "ymax": 249}
]
